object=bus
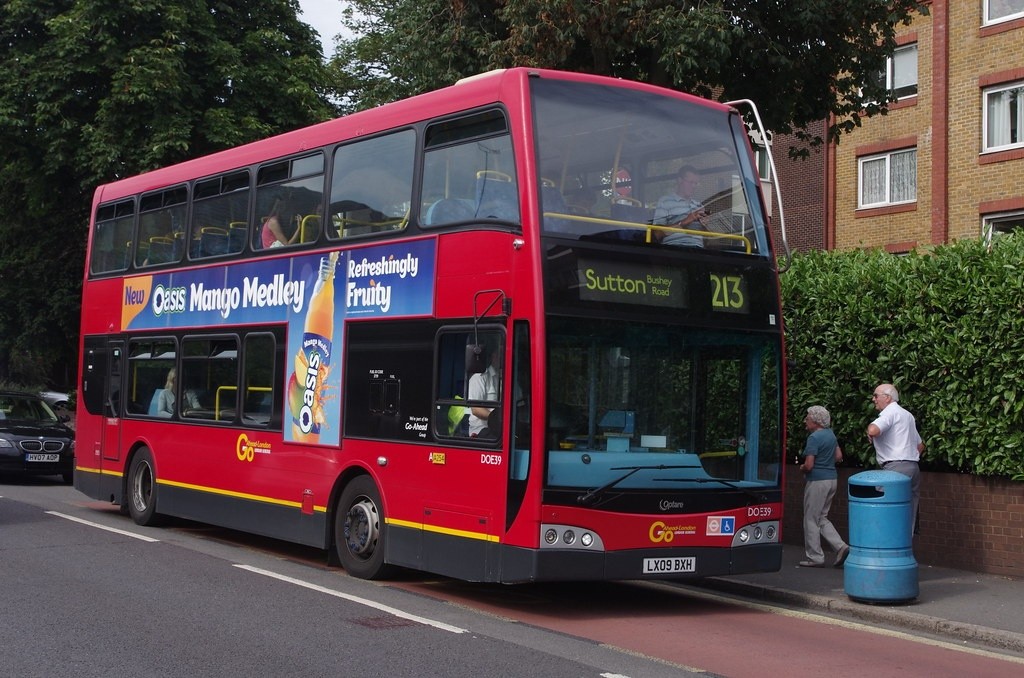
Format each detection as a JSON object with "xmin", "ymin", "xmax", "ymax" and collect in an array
[{"xmin": 73, "ymin": 68, "xmax": 797, "ymax": 590}]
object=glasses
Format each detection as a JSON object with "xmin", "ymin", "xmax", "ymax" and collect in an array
[
  {"xmin": 680, "ymin": 176, "xmax": 699, "ymax": 185},
  {"xmin": 873, "ymin": 393, "xmax": 889, "ymax": 398}
]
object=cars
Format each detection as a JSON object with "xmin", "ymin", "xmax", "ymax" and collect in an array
[
  {"xmin": 40, "ymin": 391, "xmax": 73, "ymax": 423},
  {"xmin": 0, "ymin": 390, "xmax": 74, "ymax": 483}
]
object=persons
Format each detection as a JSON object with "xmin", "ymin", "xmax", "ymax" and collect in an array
[
  {"xmin": 260, "ymin": 186, "xmax": 303, "ymax": 250},
  {"xmin": 157, "ymin": 365, "xmax": 201, "ymax": 417},
  {"xmin": 866, "ymin": 383, "xmax": 925, "ymax": 542},
  {"xmin": 566, "ymin": 189, "xmax": 604, "ymax": 233},
  {"xmin": 307, "ymin": 203, "xmax": 340, "ymax": 241},
  {"xmin": 467, "ymin": 338, "xmax": 525, "ymax": 441},
  {"xmin": 19, "ymin": 407, "xmax": 35, "ymax": 420},
  {"xmin": 651, "ymin": 164, "xmax": 711, "ymax": 247},
  {"xmin": 798, "ymin": 405, "xmax": 849, "ymax": 567}
]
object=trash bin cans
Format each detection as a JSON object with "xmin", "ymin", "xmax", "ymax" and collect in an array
[{"xmin": 843, "ymin": 470, "xmax": 919, "ymax": 604}]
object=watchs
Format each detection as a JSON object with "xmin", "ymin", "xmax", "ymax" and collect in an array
[{"xmin": 677, "ymin": 221, "xmax": 684, "ymax": 228}]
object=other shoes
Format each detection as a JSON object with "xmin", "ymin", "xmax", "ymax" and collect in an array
[
  {"xmin": 834, "ymin": 543, "xmax": 849, "ymax": 566},
  {"xmin": 800, "ymin": 558, "xmax": 825, "ymax": 568}
]
